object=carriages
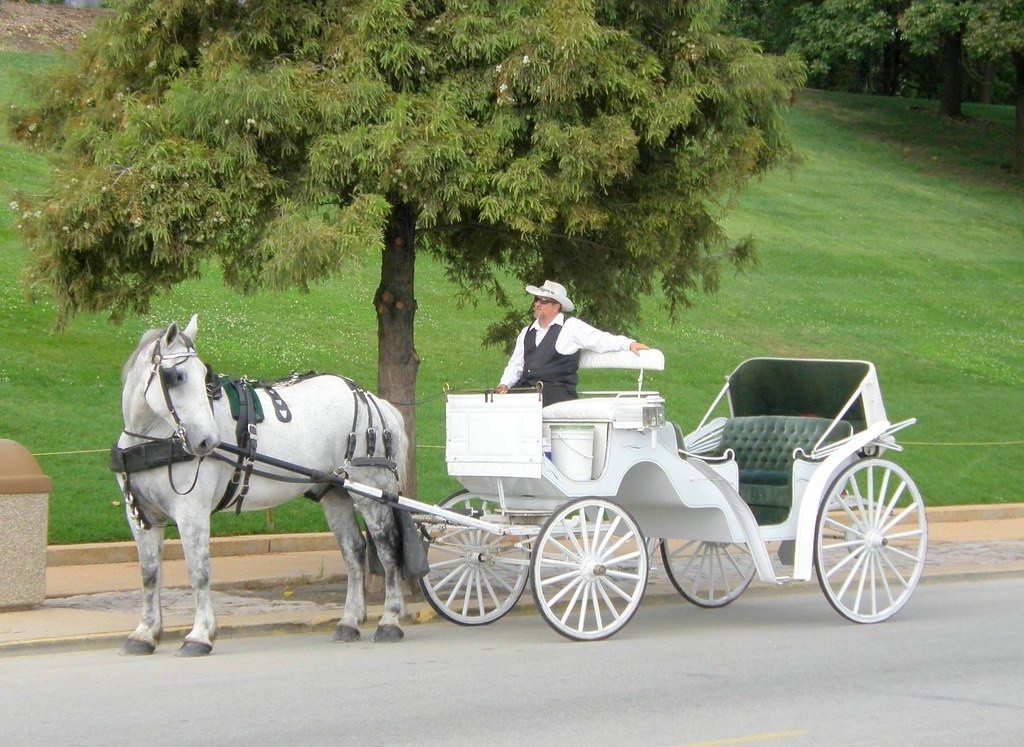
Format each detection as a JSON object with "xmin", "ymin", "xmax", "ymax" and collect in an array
[{"xmin": 109, "ymin": 313, "xmax": 929, "ymax": 657}]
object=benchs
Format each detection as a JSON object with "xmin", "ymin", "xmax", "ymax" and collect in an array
[
  {"xmin": 544, "ymin": 398, "xmax": 642, "ymax": 476},
  {"xmin": 705, "ymin": 415, "xmax": 855, "ymax": 510}
]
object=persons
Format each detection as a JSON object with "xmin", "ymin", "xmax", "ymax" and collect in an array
[{"xmin": 496, "ymin": 279, "xmax": 650, "ymax": 409}]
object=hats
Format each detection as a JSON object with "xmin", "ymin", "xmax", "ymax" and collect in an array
[{"xmin": 526, "ymin": 280, "xmax": 574, "ymax": 312}]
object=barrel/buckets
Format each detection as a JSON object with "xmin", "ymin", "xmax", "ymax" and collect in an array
[{"xmin": 548, "ymin": 425, "xmax": 595, "ymax": 480}]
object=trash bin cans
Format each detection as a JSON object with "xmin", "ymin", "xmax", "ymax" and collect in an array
[{"xmin": 0, "ymin": 438, "xmax": 52, "ymax": 611}]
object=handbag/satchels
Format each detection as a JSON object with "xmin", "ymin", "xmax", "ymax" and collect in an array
[{"xmin": 367, "ymin": 506, "xmax": 431, "ymax": 581}]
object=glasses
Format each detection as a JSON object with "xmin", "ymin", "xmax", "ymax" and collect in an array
[{"xmin": 534, "ymin": 296, "xmax": 558, "ymax": 305}]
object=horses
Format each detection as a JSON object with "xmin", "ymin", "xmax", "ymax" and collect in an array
[{"xmin": 115, "ymin": 313, "xmax": 411, "ymax": 657}]
